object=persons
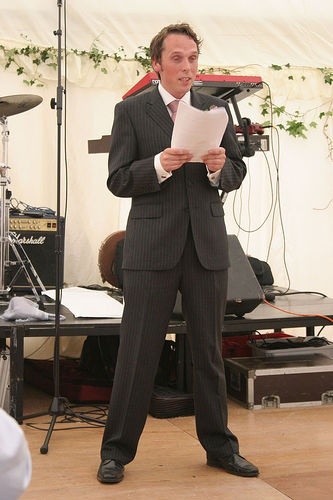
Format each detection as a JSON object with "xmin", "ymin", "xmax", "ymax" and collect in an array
[{"xmin": 96, "ymin": 23, "xmax": 259, "ymax": 485}]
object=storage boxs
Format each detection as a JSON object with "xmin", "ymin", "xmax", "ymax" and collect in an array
[{"xmin": 224, "ymin": 353, "xmax": 333, "ymax": 410}]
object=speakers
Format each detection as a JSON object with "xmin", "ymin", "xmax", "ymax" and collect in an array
[
  {"xmin": 169, "ymin": 235, "xmax": 265, "ymax": 320},
  {"xmin": 7, "ymin": 215, "xmax": 65, "ymax": 291}
]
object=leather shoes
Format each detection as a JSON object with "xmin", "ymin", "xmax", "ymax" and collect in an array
[
  {"xmin": 97, "ymin": 459, "xmax": 124, "ymax": 482},
  {"xmin": 207, "ymin": 453, "xmax": 258, "ymax": 477}
]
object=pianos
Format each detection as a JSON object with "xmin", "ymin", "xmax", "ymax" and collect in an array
[
  {"xmin": 123, "ymin": 71, "xmax": 262, "ymax": 101},
  {"xmin": 88, "ymin": 132, "xmax": 269, "ymax": 154}
]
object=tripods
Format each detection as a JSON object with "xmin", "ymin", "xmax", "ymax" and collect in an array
[{"xmin": 15, "ymin": 0, "xmax": 108, "ymax": 455}]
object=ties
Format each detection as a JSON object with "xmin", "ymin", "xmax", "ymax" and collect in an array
[{"xmin": 167, "ymin": 100, "xmax": 180, "ymax": 123}]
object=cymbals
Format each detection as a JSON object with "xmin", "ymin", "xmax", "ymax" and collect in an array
[{"xmin": 0, "ymin": 94, "xmax": 43, "ymax": 118}]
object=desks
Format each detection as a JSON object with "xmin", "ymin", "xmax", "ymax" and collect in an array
[{"xmin": 0, "ymin": 285, "xmax": 333, "ymax": 425}]
